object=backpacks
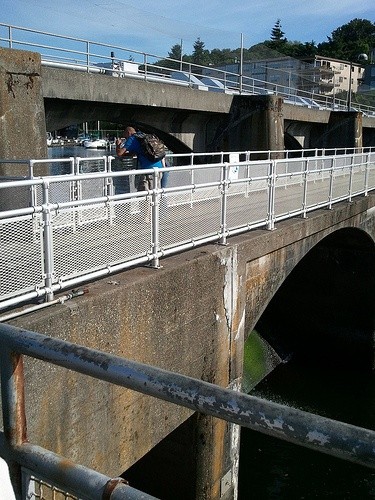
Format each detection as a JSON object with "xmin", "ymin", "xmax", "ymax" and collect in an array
[{"xmin": 135, "ymin": 133, "xmax": 166, "ymax": 162}]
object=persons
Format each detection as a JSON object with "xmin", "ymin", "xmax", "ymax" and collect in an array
[
  {"xmin": 116, "ymin": 127, "xmax": 163, "ymax": 224},
  {"xmin": 151, "ymin": 134, "xmax": 170, "ymax": 210}
]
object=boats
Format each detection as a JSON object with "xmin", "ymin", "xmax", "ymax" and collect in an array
[{"xmin": 46, "ymin": 120, "xmax": 126, "ymax": 151}]
